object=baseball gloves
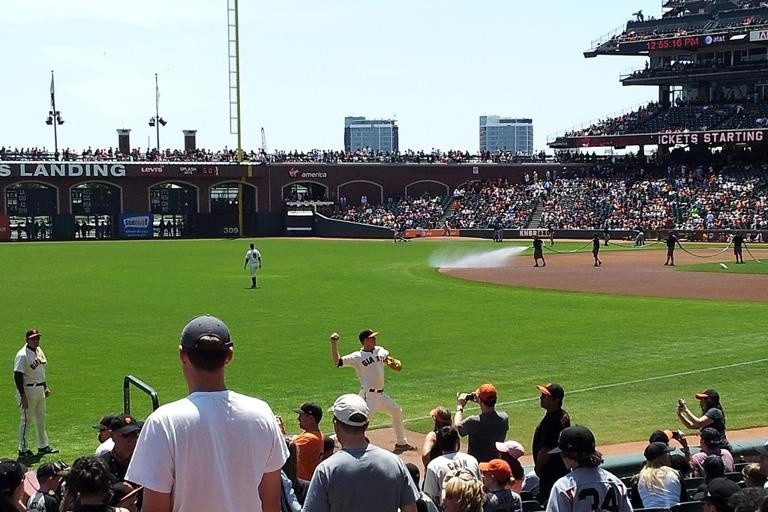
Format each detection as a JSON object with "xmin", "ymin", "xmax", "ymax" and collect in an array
[{"xmin": 386, "ymin": 358, "xmax": 401, "ymax": 370}]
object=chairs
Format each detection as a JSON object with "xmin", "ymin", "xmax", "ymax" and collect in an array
[
  {"xmin": 636, "ymin": 105, "xmax": 768, "ymax": 135},
  {"xmin": 517, "ymin": 461, "xmax": 768, "ymax": 512}
]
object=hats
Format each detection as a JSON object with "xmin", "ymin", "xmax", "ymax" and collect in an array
[
  {"xmin": 292, "ymin": 402, "xmax": 322, "ymax": 416},
  {"xmin": 495, "ymin": 441, "xmax": 525, "ymax": 458},
  {"xmin": 644, "ymin": 430, "xmax": 675, "ymax": 460},
  {"xmin": 536, "ymin": 381, "xmax": 564, "ymax": 401},
  {"xmin": 431, "ymin": 410, "xmax": 450, "ymax": 423},
  {"xmin": 328, "ymin": 393, "xmax": 369, "ymax": 427},
  {"xmin": 753, "ymin": 445, "xmax": 768, "ymax": 456},
  {"xmin": 179, "ymin": 314, "xmax": 233, "ymax": 352},
  {"xmin": 360, "ymin": 329, "xmax": 379, "ymax": 342},
  {"xmin": 702, "ymin": 428, "xmax": 723, "ymax": 444},
  {"xmin": 548, "ymin": 426, "xmax": 594, "ymax": 454},
  {"xmin": 694, "ymin": 478, "xmax": 743, "ymax": 500},
  {"xmin": 695, "ymin": 389, "xmax": 720, "ymax": 403},
  {"xmin": 37, "ymin": 462, "xmax": 61, "ymax": 478},
  {"xmin": 703, "ymin": 454, "xmax": 725, "ymax": 474},
  {"xmin": 110, "ymin": 482, "xmax": 144, "ymax": 506},
  {"xmin": 476, "ymin": 385, "xmax": 496, "ymax": 401},
  {"xmin": 0, "ymin": 459, "xmax": 27, "ymax": 492},
  {"xmin": 480, "ymin": 459, "xmax": 512, "ymax": 480},
  {"xmin": 26, "ymin": 330, "xmax": 41, "ymax": 339},
  {"xmin": 93, "ymin": 413, "xmax": 145, "ymax": 434}
]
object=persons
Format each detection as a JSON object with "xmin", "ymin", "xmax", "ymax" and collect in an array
[
  {"xmin": 12, "ymin": 216, "xmax": 49, "ymax": 241},
  {"xmin": 442, "ymin": 169, "xmax": 545, "ymax": 242},
  {"xmin": 565, "ymin": 93, "xmax": 767, "ymax": 137},
  {"xmin": 625, "ymin": 55, "xmax": 745, "ymax": 79},
  {"xmin": 152, "ymin": 215, "xmax": 182, "ymax": 238},
  {"xmin": 75, "ymin": 218, "xmax": 111, "ymax": 239},
  {"xmin": 284, "ymin": 187, "xmax": 329, "ymax": 213},
  {"xmin": 330, "ymin": 193, "xmax": 444, "ymax": 243},
  {"xmin": 612, "ymin": 1, "xmax": 767, "ymax": 44},
  {"xmin": 213, "ymin": 197, "xmax": 238, "ymax": 210},
  {"xmin": 1, "ymin": 315, "xmax": 767, "ymax": 512},
  {"xmin": 537, "ymin": 148, "xmax": 767, "ymax": 243},
  {"xmin": 0, "ymin": 146, "xmax": 549, "ymax": 163}
]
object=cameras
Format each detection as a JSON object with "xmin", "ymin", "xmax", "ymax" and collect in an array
[
  {"xmin": 672, "ymin": 429, "xmax": 684, "ymax": 440},
  {"xmin": 466, "ymin": 392, "xmax": 474, "ymax": 401}
]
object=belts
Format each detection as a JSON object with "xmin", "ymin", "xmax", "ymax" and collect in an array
[
  {"xmin": 27, "ymin": 381, "xmax": 46, "ymax": 387},
  {"xmin": 369, "ymin": 388, "xmax": 384, "ymax": 393}
]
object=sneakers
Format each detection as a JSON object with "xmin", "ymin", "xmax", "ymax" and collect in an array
[
  {"xmin": 395, "ymin": 443, "xmax": 417, "ymax": 453},
  {"xmin": 38, "ymin": 445, "xmax": 59, "ymax": 453},
  {"xmin": 18, "ymin": 448, "xmax": 34, "ymax": 457}
]
